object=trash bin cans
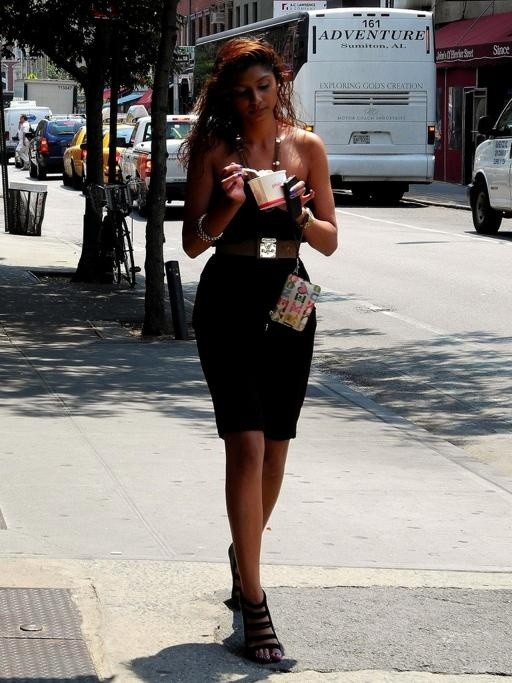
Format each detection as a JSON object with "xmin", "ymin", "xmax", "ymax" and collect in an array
[{"xmin": 6, "ymin": 180, "xmax": 49, "ymax": 237}]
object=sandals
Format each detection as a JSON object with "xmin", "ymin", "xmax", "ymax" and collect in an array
[
  {"xmin": 239, "ymin": 589, "xmax": 282, "ymax": 664},
  {"xmin": 228, "ymin": 543, "xmax": 242, "ymax": 602}
]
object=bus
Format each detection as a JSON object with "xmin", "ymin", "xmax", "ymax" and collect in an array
[{"xmin": 191, "ymin": 5, "xmax": 442, "ymax": 204}]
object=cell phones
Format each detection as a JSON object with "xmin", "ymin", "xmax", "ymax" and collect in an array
[{"xmin": 283, "ymin": 174, "xmax": 304, "ymax": 221}]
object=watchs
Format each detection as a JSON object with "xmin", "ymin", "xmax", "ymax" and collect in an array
[{"xmin": 295, "ymin": 206, "xmax": 316, "ymax": 230}]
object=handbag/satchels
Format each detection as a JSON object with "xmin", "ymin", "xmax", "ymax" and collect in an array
[{"xmin": 271, "ymin": 274, "xmax": 321, "ymax": 333}]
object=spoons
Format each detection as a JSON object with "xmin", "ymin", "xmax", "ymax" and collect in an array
[{"xmin": 232, "ymin": 167, "xmax": 274, "ymax": 177}]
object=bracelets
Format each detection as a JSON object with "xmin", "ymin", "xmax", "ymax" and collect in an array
[{"xmin": 195, "ymin": 211, "xmax": 226, "ymax": 244}]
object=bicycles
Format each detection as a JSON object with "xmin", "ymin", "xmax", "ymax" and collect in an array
[{"xmin": 79, "ymin": 172, "xmax": 146, "ymax": 291}]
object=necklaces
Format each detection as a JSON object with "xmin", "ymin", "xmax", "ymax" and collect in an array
[{"xmin": 226, "ymin": 116, "xmax": 282, "ymax": 214}]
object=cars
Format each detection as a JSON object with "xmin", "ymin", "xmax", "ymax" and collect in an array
[{"xmin": 0, "ymin": 98, "xmax": 201, "ymax": 215}]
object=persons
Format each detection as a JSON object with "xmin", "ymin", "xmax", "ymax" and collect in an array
[
  {"xmin": 178, "ymin": 34, "xmax": 339, "ymax": 665},
  {"xmin": 15, "ymin": 112, "xmax": 32, "ymax": 169}
]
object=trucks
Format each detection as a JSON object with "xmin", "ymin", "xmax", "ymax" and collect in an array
[{"xmin": 465, "ymin": 92, "xmax": 512, "ymax": 238}]
